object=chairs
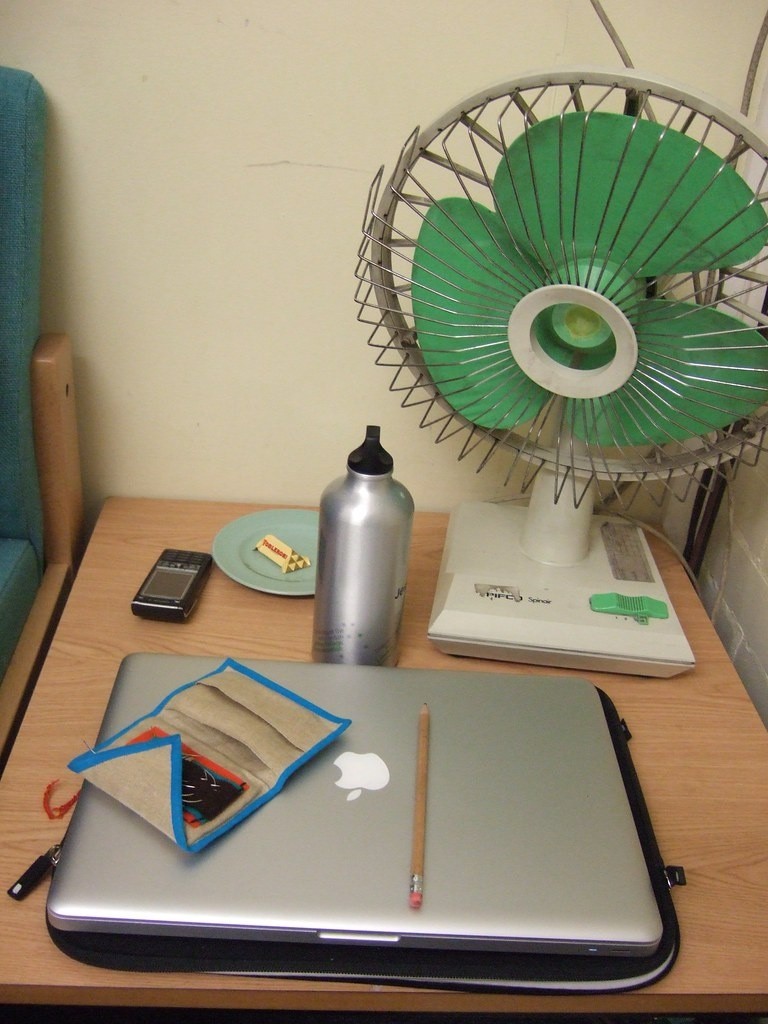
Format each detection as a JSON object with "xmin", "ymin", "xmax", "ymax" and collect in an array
[{"xmin": 0, "ymin": 66, "xmax": 88, "ymax": 761}]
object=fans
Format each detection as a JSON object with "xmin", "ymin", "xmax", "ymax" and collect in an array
[{"xmin": 353, "ymin": 70, "xmax": 768, "ymax": 679}]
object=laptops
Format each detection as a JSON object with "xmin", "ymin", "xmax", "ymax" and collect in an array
[{"xmin": 48, "ymin": 653, "xmax": 664, "ymax": 956}]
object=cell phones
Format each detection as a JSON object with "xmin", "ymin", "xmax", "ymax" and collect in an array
[{"xmin": 132, "ymin": 549, "xmax": 213, "ymax": 623}]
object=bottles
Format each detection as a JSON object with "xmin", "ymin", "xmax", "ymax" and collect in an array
[{"xmin": 311, "ymin": 426, "xmax": 414, "ymax": 667}]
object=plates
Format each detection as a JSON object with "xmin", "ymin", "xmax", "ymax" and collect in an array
[{"xmin": 213, "ymin": 509, "xmax": 324, "ymax": 596}]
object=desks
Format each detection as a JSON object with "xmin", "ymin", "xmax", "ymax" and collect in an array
[{"xmin": 0, "ymin": 498, "xmax": 768, "ymax": 1013}]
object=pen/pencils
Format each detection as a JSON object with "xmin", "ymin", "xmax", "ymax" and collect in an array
[{"xmin": 409, "ymin": 702, "xmax": 430, "ymax": 908}]
